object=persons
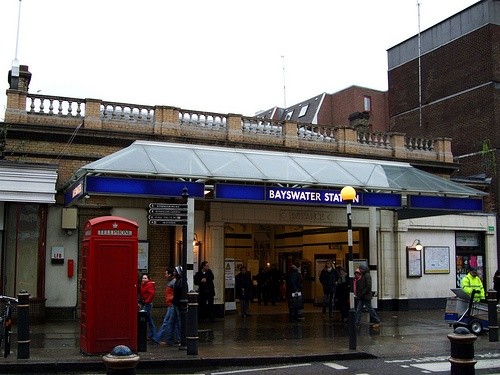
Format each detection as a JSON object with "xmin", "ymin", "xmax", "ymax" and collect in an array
[
  {"xmin": 493, "ymin": 268, "xmax": 500, "ymax": 305},
  {"xmin": 235, "ymin": 266, "xmax": 252, "ymax": 318},
  {"xmin": 460, "ymin": 267, "xmax": 486, "ymax": 303},
  {"xmin": 319, "ymin": 259, "xmax": 340, "ymax": 318},
  {"xmin": 140, "ymin": 275, "xmax": 158, "ymax": 345},
  {"xmin": 167, "ymin": 265, "xmax": 188, "ymax": 346},
  {"xmin": 350, "ymin": 268, "xmax": 363, "ymax": 314},
  {"xmin": 152, "ymin": 268, "xmax": 177, "ymax": 345},
  {"xmin": 193, "ymin": 261, "xmax": 216, "ymax": 323},
  {"xmin": 352, "ymin": 264, "xmax": 381, "ymax": 327},
  {"xmin": 283, "ymin": 264, "xmax": 304, "ymax": 323},
  {"xmin": 255, "ymin": 264, "xmax": 283, "ymax": 306},
  {"xmin": 335, "ymin": 265, "xmax": 350, "ymax": 312}
]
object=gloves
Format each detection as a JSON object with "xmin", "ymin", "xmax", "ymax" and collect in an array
[
  {"xmin": 474, "ymin": 290, "xmax": 481, "ymax": 294},
  {"xmin": 480, "ymin": 299, "xmax": 486, "ymax": 302}
]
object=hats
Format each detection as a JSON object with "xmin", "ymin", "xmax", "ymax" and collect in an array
[
  {"xmin": 175, "ymin": 265, "xmax": 183, "ymax": 276},
  {"xmin": 469, "ymin": 267, "xmax": 477, "ymax": 272},
  {"xmin": 354, "ymin": 268, "xmax": 362, "ymax": 275}
]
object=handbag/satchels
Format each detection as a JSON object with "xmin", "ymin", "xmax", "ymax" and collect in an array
[{"xmin": 289, "ymin": 293, "xmax": 305, "ymax": 310}]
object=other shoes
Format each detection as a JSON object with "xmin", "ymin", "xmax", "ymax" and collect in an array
[
  {"xmin": 338, "ymin": 317, "xmax": 349, "ymax": 322},
  {"xmin": 372, "ymin": 321, "xmax": 382, "ymax": 328},
  {"xmin": 295, "ymin": 315, "xmax": 305, "ymax": 320},
  {"xmin": 240, "ymin": 314, "xmax": 244, "ymax": 318},
  {"xmin": 289, "ymin": 317, "xmax": 301, "ymax": 323},
  {"xmin": 329, "ymin": 312, "xmax": 334, "ymax": 317},
  {"xmin": 245, "ymin": 314, "xmax": 251, "ymax": 319},
  {"xmin": 322, "ymin": 308, "xmax": 327, "ymax": 313}
]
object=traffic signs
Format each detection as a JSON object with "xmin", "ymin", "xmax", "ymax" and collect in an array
[
  {"xmin": 148, "ymin": 215, "xmax": 188, "ymax": 219},
  {"xmin": 148, "ymin": 219, "xmax": 187, "ymax": 226},
  {"xmin": 149, "ymin": 203, "xmax": 188, "ymax": 209},
  {"xmin": 149, "ymin": 209, "xmax": 188, "ymax": 214}
]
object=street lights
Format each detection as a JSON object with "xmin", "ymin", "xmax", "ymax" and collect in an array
[{"xmin": 340, "ymin": 185, "xmax": 357, "ymax": 351}]
